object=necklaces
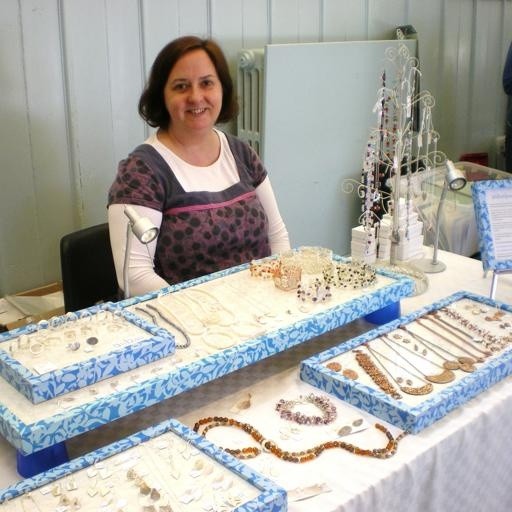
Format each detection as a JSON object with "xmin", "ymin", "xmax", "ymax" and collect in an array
[
  {"xmin": 433, "ymin": 313, "xmax": 500, "ymax": 353},
  {"xmin": 133, "ymin": 296, "xmax": 192, "ymax": 350},
  {"xmin": 397, "ymin": 323, "xmax": 477, "ymax": 375},
  {"xmin": 442, "ymin": 309, "xmax": 508, "ymax": 349},
  {"xmin": 415, "ymin": 319, "xmax": 484, "ymax": 363},
  {"xmin": 426, "ymin": 315, "xmax": 493, "ymax": 357},
  {"xmin": 376, "ymin": 333, "xmax": 455, "ymax": 385},
  {"xmin": 272, "ymin": 393, "xmax": 338, "ymax": 424},
  {"xmin": 190, "ymin": 413, "xmax": 410, "ymax": 470},
  {"xmin": 185, "ymin": 286, "xmax": 264, "ymax": 338},
  {"xmin": 157, "ymin": 293, "xmax": 234, "ymax": 349},
  {"xmin": 363, "ymin": 341, "xmax": 433, "ymax": 397}
]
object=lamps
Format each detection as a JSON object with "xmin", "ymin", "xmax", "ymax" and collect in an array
[{"xmin": 410, "ymin": 160, "xmax": 467, "ymax": 273}]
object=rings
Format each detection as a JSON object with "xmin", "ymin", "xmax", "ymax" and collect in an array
[
  {"xmin": 66, "ymin": 313, "xmax": 81, "ymax": 329},
  {"xmin": 96, "ymin": 309, "xmax": 105, "ymax": 320},
  {"xmin": 18, "ymin": 335, "xmax": 31, "ymax": 346},
  {"xmin": 114, "ymin": 309, "xmax": 124, "ymax": 319},
  {"xmin": 79, "ymin": 310, "xmax": 92, "ymax": 325},
  {"xmin": 28, "ymin": 341, "xmax": 43, "ymax": 354},
  {"xmin": 36, "ymin": 319, "xmax": 50, "ymax": 336},
  {"xmin": 49, "ymin": 315, "xmax": 62, "ymax": 330}
]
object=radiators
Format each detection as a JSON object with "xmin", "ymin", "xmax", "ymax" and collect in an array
[{"xmin": 237, "ymin": 49, "xmax": 264, "ymax": 156}]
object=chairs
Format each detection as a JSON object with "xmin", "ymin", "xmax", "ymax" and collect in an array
[
  {"xmin": 489, "ymin": 136, "xmax": 506, "ymax": 171},
  {"xmin": 60, "ymin": 222, "xmax": 124, "ymax": 314},
  {"xmin": 364, "ymin": 157, "xmax": 426, "ymax": 226}
]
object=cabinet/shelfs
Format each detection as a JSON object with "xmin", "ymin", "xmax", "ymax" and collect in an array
[{"xmin": 0, "ymin": 247, "xmax": 512, "ymax": 512}]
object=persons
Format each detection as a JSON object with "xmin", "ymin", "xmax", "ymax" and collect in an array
[
  {"xmin": 107, "ymin": 36, "xmax": 292, "ymax": 296},
  {"xmin": 501, "ymin": 37, "xmax": 511, "ymax": 176}
]
